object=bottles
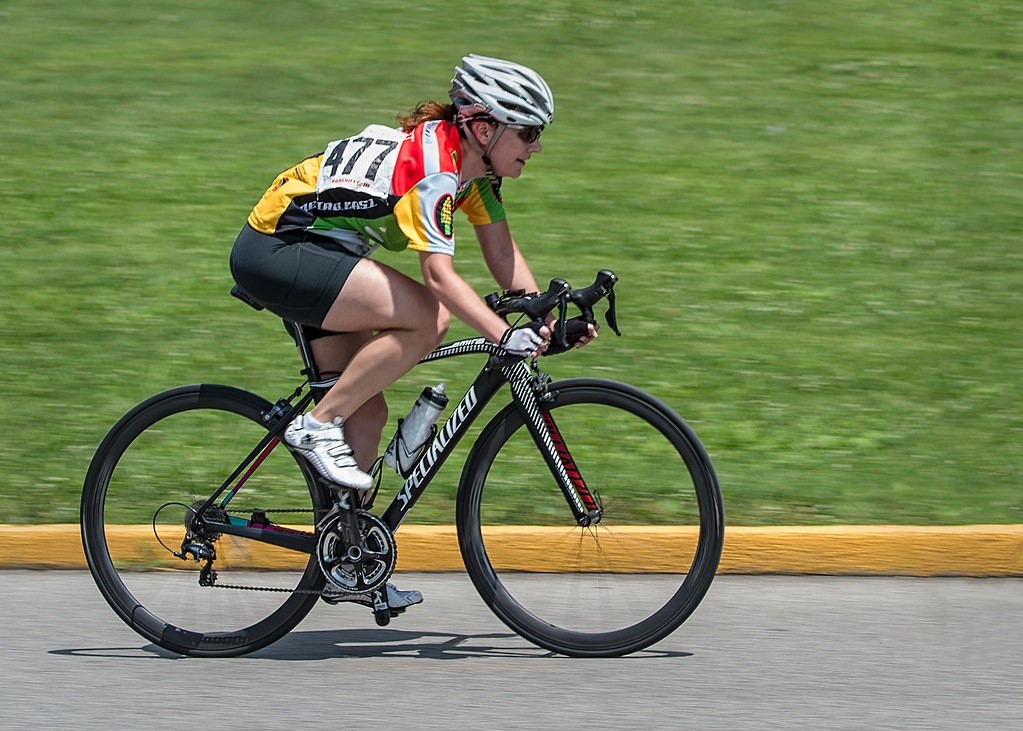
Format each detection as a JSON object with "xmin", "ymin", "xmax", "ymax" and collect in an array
[{"xmin": 383, "ymin": 382, "xmax": 450, "ymax": 473}]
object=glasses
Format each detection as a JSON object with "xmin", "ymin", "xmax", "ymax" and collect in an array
[{"xmin": 515, "ymin": 125, "xmax": 541, "ymax": 143}]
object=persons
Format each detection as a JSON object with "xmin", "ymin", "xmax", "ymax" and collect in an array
[{"xmin": 229, "ymin": 53, "xmax": 598, "ymax": 608}]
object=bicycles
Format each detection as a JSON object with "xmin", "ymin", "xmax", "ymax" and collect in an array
[{"xmin": 80, "ymin": 270, "xmax": 724, "ymax": 660}]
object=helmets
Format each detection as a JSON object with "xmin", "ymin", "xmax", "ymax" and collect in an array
[{"xmin": 447, "ymin": 53, "xmax": 555, "ymax": 126}]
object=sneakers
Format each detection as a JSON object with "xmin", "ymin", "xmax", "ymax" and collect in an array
[
  {"xmin": 320, "ymin": 574, "xmax": 423, "ymax": 610},
  {"xmin": 283, "ymin": 413, "xmax": 374, "ymax": 491}
]
